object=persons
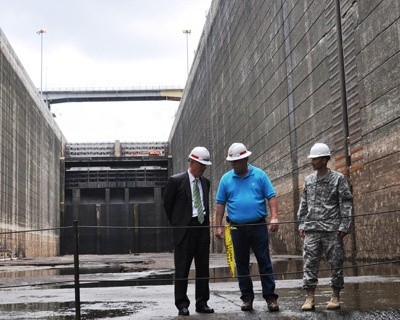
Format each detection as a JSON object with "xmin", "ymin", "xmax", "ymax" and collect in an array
[
  {"xmin": 215, "ymin": 143, "xmax": 279, "ymax": 311},
  {"xmin": 163, "ymin": 146, "xmax": 214, "ymax": 316},
  {"xmin": 296, "ymin": 143, "xmax": 354, "ymax": 311}
]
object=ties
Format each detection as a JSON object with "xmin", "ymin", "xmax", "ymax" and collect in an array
[{"xmin": 193, "ymin": 178, "xmax": 204, "ymax": 224}]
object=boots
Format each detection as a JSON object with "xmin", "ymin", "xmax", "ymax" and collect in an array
[
  {"xmin": 300, "ymin": 288, "xmax": 315, "ymax": 311},
  {"xmin": 327, "ymin": 287, "xmax": 340, "ymax": 309}
]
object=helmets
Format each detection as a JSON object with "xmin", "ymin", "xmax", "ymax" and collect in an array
[
  {"xmin": 307, "ymin": 143, "xmax": 332, "ymax": 159},
  {"xmin": 226, "ymin": 143, "xmax": 252, "ymax": 161},
  {"xmin": 189, "ymin": 147, "xmax": 212, "ymax": 165}
]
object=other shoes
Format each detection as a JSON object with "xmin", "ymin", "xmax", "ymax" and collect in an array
[
  {"xmin": 266, "ymin": 299, "xmax": 278, "ymax": 311},
  {"xmin": 178, "ymin": 307, "xmax": 190, "ymax": 315},
  {"xmin": 195, "ymin": 303, "xmax": 213, "ymax": 313},
  {"xmin": 241, "ymin": 299, "xmax": 253, "ymax": 310}
]
